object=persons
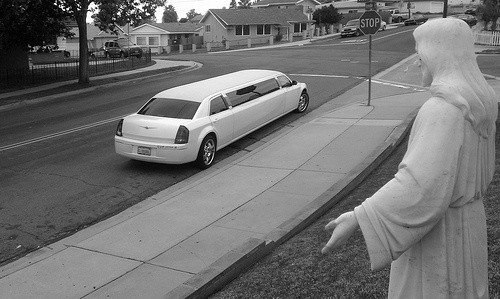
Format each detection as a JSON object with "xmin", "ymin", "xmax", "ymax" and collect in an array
[{"xmin": 320, "ymin": 17, "xmax": 500, "ymax": 299}]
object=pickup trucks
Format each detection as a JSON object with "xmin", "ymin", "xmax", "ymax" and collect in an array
[{"xmin": 387, "ymin": 9, "xmax": 408, "ymax": 22}]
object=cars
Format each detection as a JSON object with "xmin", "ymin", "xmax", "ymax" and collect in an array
[
  {"xmin": 340, "ymin": 19, "xmax": 359, "ymax": 38},
  {"xmin": 457, "ymin": 14, "xmax": 477, "ymax": 26},
  {"xmin": 114, "ymin": 69, "xmax": 310, "ymax": 170},
  {"xmin": 28, "ymin": 45, "xmax": 71, "ymax": 59},
  {"xmin": 380, "ymin": 20, "xmax": 386, "ymax": 31},
  {"xmin": 465, "ymin": 8, "xmax": 476, "ymax": 14},
  {"xmin": 404, "ymin": 14, "xmax": 429, "ymax": 26},
  {"xmin": 91, "ymin": 46, "xmax": 106, "ymax": 59}
]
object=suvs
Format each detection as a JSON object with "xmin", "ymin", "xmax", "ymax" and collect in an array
[{"xmin": 104, "ymin": 38, "xmax": 143, "ymax": 59}]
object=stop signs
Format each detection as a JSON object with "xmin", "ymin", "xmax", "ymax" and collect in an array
[{"xmin": 358, "ymin": 11, "xmax": 381, "ymax": 36}]
